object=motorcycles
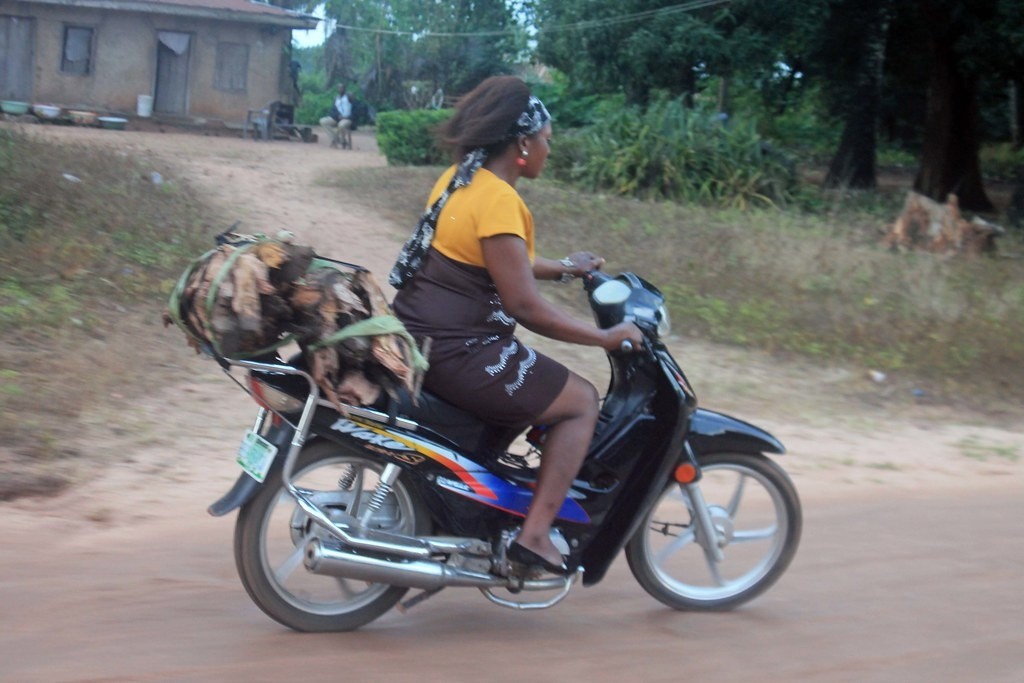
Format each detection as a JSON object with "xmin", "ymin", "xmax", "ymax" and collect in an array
[{"xmin": 207, "ymin": 268, "xmax": 802, "ymax": 632}]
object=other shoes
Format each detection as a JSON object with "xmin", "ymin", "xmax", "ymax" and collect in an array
[{"xmin": 504, "ymin": 540, "xmax": 570, "ymax": 575}]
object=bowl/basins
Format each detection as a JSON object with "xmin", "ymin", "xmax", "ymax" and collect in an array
[
  {"xmin": 97, "ymin": 116, "xmax": 128, "ymax": 129},
  {"xmin": 0, "ymin": 100, "xmax": 32, "ymax": 114},
  {"xmin": 68, "ymin": 110, "xmax": 99, "ymax": 125},
  {"xmin": 32, "ymin": 105, "xmax": 62, "ymax": 119}
]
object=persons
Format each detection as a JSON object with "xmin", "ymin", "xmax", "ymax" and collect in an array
[
  {"xmin": 388, "ymin": 74, "xmax": 644, "ymax": 576},
  {"xmin": 319, "ymin": 83, "xmax": 362, "ymax": 148}
]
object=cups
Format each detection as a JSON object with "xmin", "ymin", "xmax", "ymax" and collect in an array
[{"xmin": 137, "ymin": 94, "xmax": 154, "ymax": 117}]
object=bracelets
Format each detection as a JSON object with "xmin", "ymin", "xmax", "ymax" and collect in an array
[{"xmin": 558, "ymin": 259, "xmax": 575, "ymax": 284}]
